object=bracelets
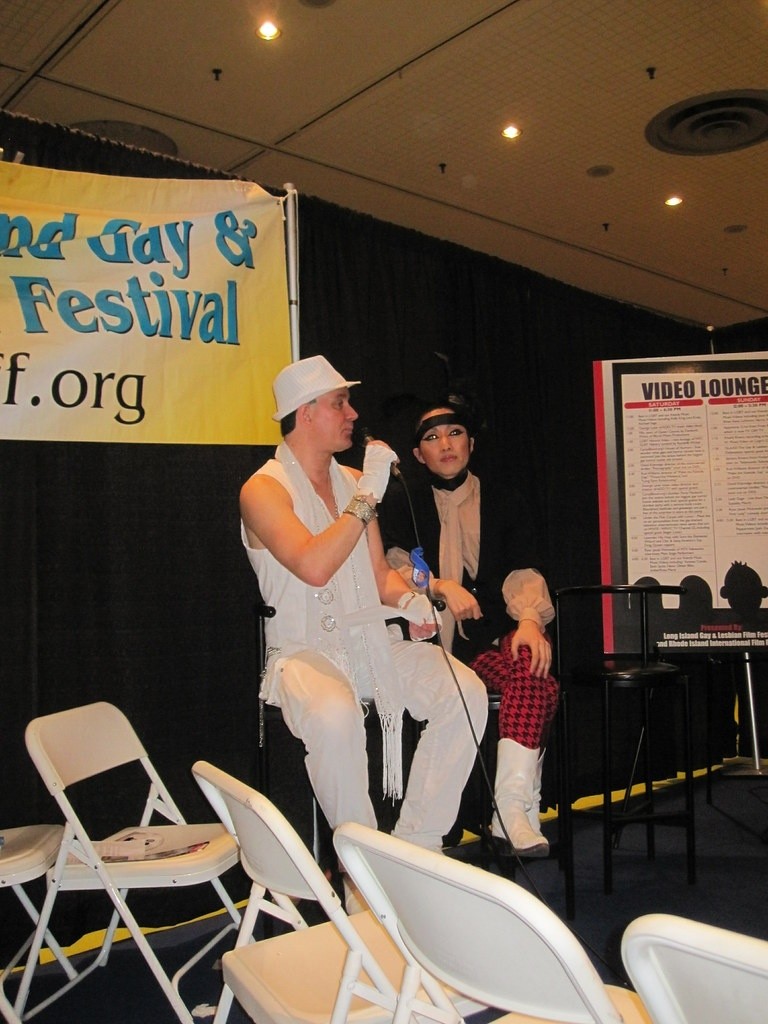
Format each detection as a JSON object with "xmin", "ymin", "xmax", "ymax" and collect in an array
[
  {"xmin": 343, "ymin": 494, "xmax": 378, "ymax": 526},
  {"xmin": 397, "ymin": 591, "xmax": 417, "ymax": 610}
]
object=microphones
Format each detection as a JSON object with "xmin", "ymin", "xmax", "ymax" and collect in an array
[{"xmin": 351, "ymin": 427, "xmax": 403, "ymax": 480}]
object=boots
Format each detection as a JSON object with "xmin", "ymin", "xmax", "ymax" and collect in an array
[{"xmin": 488, "ymin": 737, "xmax": 550, "ymax": 857}]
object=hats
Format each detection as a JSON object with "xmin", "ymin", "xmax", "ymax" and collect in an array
[{"xmin": 272, "ymin": 355, "xmax": 361, "ymax": 422}]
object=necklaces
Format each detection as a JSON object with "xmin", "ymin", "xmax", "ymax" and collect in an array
[{"xmin": 334, "ymin": 502, "xmax": 338, "ymax": 518}]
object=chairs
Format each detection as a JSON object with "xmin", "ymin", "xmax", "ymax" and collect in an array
[
  {"xmin": 244, "ymin": 584, "xmax": 698, "ymax": 940},
  {"xmin": 191, "ymin": 761, "xmax": 491, "ymax": 1024},
  {"xmin": 333, "ymin": 823, "xmax": 655, "ymax": 1024},
  {"xmin": 620, "ymin": 913, "xmax": 768, "ymax": 1024},
  {"xmin": 0, "ymin": 825, "xmax": 80, "ymax": 1024},
  {"xmin": 13, "ymin": 702, "xmax": 257, "ymax": 1024}
]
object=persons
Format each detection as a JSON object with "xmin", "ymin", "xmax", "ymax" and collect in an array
[
  {"xmin": 383, "ymin": 386, "xmax": 560, "ymax": 857},
  {"xmin": 239, "ymin": 354, "xmax": 488, "ymax": 915}
]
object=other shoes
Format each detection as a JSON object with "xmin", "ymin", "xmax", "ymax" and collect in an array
[{"xmin": 343, "ymin": 877, "xmax": 370, "ymax": 916}]
